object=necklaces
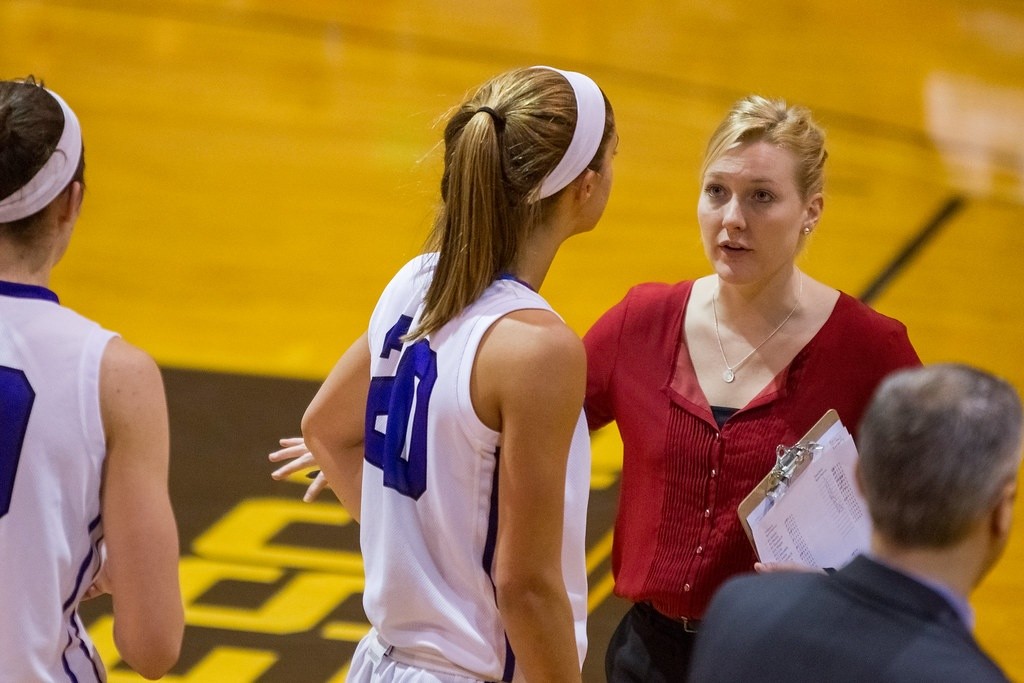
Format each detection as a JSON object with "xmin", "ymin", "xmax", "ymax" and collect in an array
[{"xmin": 712, "ymin": 265, "xmax": 803, "ymax": 383}]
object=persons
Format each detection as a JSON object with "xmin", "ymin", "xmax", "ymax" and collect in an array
[
  {"xmin": 301, "ymin": 65, "xmax": 620, "ymax": 682},
  {"xmin": 685, "ymin": 359, "xmax": 1024, "ymax": 683},
  {"xmin": 268, "ymin": 95, "xmax": 924, "ymax": 682},
  {"xmin": 1, "ymin": 74, "xmax": 185, "ymax": 681}
]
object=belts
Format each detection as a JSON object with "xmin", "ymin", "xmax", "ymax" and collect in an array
[{"xmin": 634, "ymin": 601, "xmax": 696, "ymax": 648}]
